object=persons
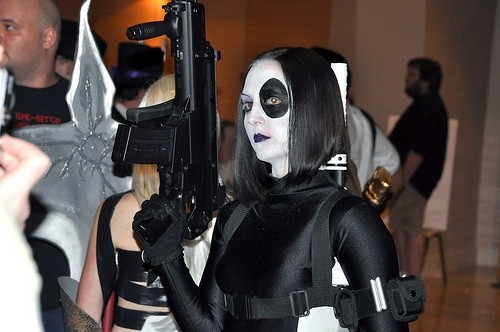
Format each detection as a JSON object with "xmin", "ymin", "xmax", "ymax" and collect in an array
[
  {"xmin": 388, "ymin": 58, "xmax": 449, "ymax": 275},
  {"xmin": 310, "ymin": 47, "xmax": 400, "ymax": 192},
  {"xmin": 132, "ymin": 47, "xmax": 410, "ymax": 332},
  {"xmin": 55, "ymin": 19, "xmax": 107, "ymax": 80},
  {"xmin": 110, "ymin": 42, "xmax": 165, "ymax": 125},
  {"xmin": 0, "ymin": 134, "xmax": 52, "ymax": 332},
  {"xmin": 0, "ymin": 0, "xmax": 71, "ymax": 137},
  {"xmin": 77, "ymin": 74, "xmax": 234, "ymax": 332}
]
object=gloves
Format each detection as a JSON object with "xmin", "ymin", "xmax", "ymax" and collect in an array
[{"xmin": 133, "ymin": 193, "xmax": 184, "ymax": 266}]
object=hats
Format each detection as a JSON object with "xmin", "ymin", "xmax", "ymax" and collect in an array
[
  {"xmin": 57, "ymin": 19, "xmax": 107, "ymax": 61},
  {"xmin": 111, "ymin": 42, "xmax": 164, "ymax": 84}
]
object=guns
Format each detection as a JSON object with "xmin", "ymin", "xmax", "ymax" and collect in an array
[{"xmin": 112, "ymin": 0, "xmax": 227, "ymax": 240}]
